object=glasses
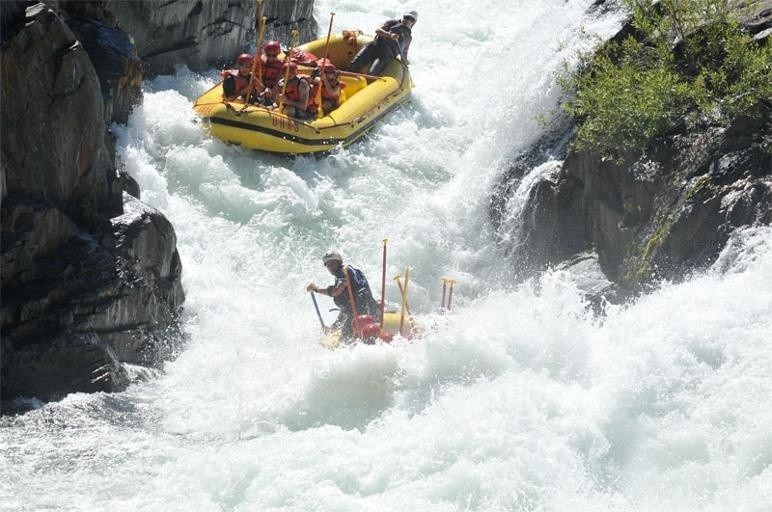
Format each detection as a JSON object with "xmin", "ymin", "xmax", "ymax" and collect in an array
[
  {"xmin": 265, "ymin": 52, "xmax": 276, "ymax": 57},
  {"xmin": 240, "ymin": 63, "xmax": 251, "ymax": 68}
]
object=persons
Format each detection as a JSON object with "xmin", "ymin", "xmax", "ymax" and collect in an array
[
  {"xmin": 344, "ymin": 9, "xmax": 419, "ymax": 85},
  {"xmin": 309, "ymin": 57, "xmax": 331, "ymax": 86},
  {"xmin": 220, "ymin": 53, "xmax": 276, "ymax": 112},
  {"xmin": 254, "ymin": 40, "xmax": 289, "ymax": 102},
  {"xmin": 305, "ymin": 248, "xmax": 382, "ymax": 343},
  {"xmin": 260, "ymin": 60, "xmax": 318, "ymax": 123},
  {"xmin": 313, "ymin": 64, "xmax": 342, "ymax": 118}
]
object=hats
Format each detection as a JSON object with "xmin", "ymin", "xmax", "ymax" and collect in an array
[{"xmin": 403, "ymin": 8, "xmax": 419, "ymax": 21}]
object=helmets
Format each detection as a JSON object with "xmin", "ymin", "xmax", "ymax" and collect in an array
[
  {"xmin": 322, "ymin": 250, "xmax": 343, "ymax": 265},
  {"xmin": 319, "ymin": 63, "xmax": 335, "ymax": 74},
  {"xmin": 281, "ymin": 62, "xmax": 298, "ymax": 75},
  {"xmin": 264, "ymin": 41, "xmax": 281, "ymax": 52},
  {"xmin": 237, "ymin": 53, "xmax": 255, "ymax": 64}
]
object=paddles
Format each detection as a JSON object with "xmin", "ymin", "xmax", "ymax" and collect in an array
[
  {"xmin": 314, "ymin": 12, "xmax": 335, "ymax": 118},
  {"xmin": 276, "ymin": 30, "xmax": 298, "ymax": 114}
]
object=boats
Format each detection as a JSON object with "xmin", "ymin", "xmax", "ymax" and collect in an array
[
  {"xmin": 192, "ymin": 31, "xmax": 414, "ymax": 157},
  {"xmin": 317, "ymin": 314, "xmax": 428, "ymax": 350}
]
